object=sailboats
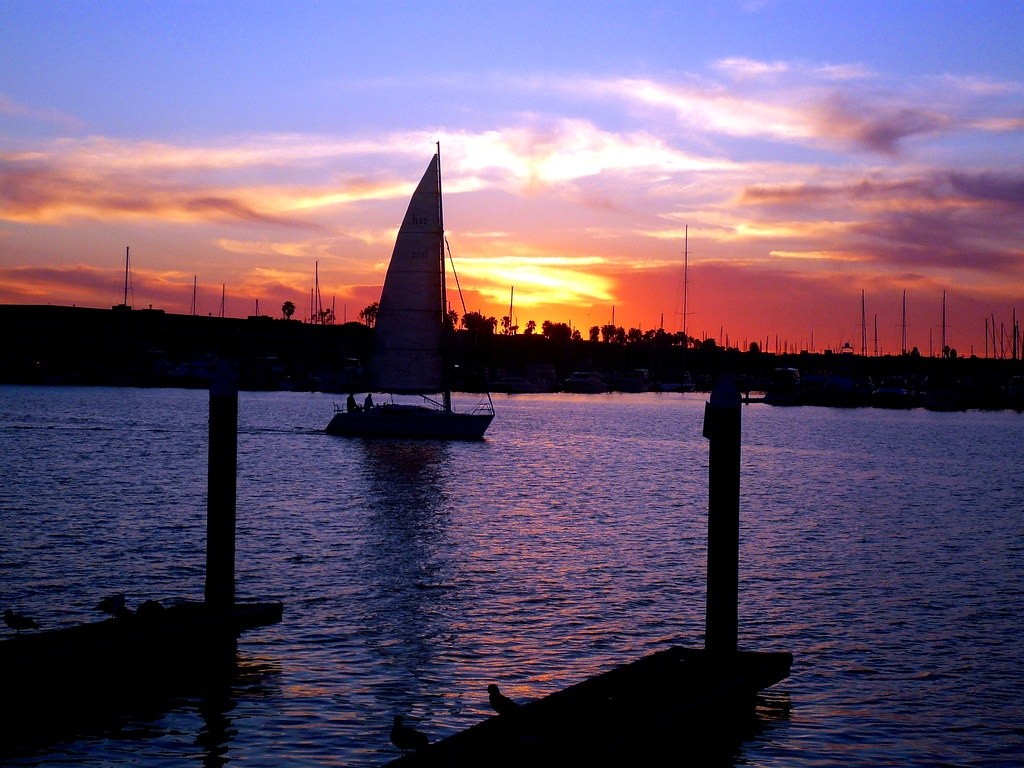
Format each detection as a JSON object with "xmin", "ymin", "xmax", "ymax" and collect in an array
[
  {"xmin": 111, "ymin": 222, "xmax": 1023, "ymax": 411},
  {"xmin": 324, "ymin": 139, "xmax": 497, "ymax": 441}
]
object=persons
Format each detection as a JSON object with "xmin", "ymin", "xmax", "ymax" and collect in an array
[
  {"xmin": 346, "ymin": 388, "xmax": 358, "ymax": 412},
  {"xmin": 364, "ymin": 394, "xmax": 375, "ymax": 410}
]
